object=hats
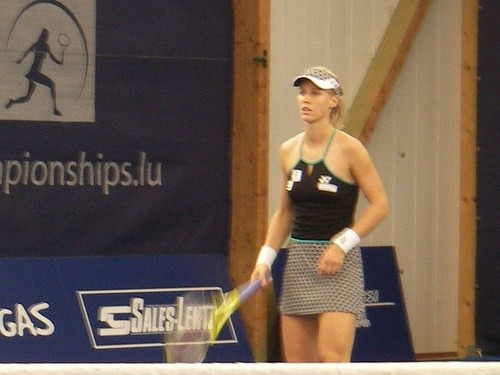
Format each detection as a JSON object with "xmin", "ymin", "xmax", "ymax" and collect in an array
[{"xmin": 294, "ymin": 66, "xmax": 341, "ymax": 94}]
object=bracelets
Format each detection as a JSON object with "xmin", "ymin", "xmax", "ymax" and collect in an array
[
  {"xmin": 330, "ymin": 227, "xmax": 361, "ymax": 255},
  {"xmin": 256, "ymin": 244, "xmax": 278, "ymax": 270}
]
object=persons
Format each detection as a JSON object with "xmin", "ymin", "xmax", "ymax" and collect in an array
[{"xmin": 250, "ymin": 66, "xmax": 389, "ymax": 363}]
